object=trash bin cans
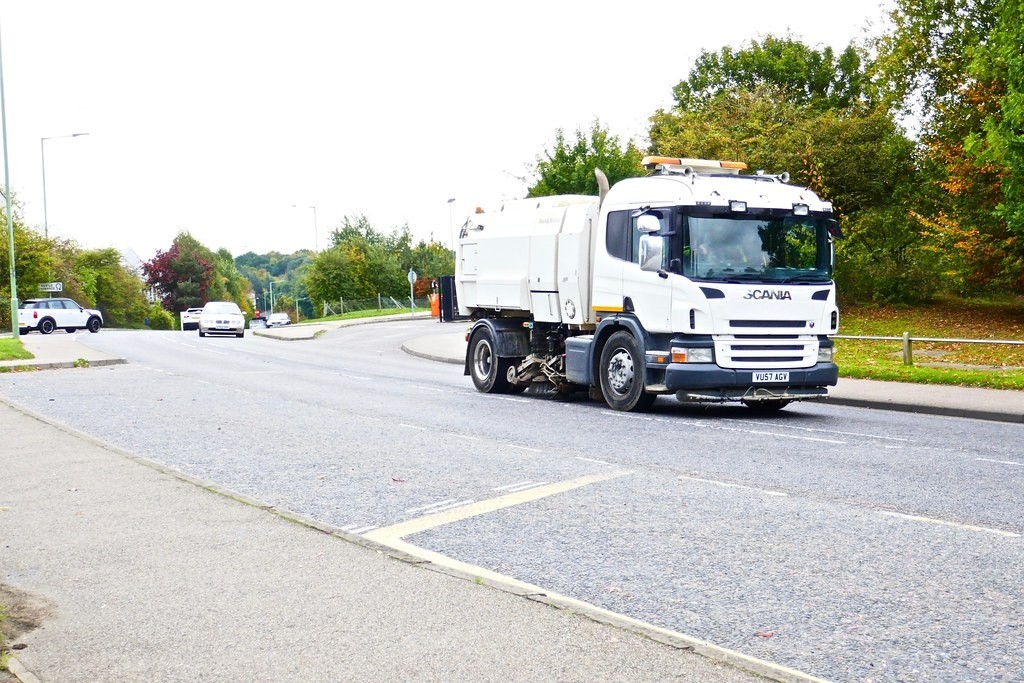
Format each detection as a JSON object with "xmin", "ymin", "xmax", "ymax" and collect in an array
[{"xmin": 430, "ymin": 294, "xmax": 440, "ymax": 316}]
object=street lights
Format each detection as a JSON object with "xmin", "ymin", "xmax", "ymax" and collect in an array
[
  {"xmin": 41, "ymin": 132, "xmax": 90, "ymax": 299},
  {"xmin": 273, "ymin": 293, "xmax": 283, "ymax": 307}
]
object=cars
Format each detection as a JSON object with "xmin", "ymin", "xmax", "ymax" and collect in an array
[
  {"xmin": 249, "ymin": 317, "xmax": 269, "ymax": 328},
  {"xmin": 194, "ymin": 301, "xmax": 246, "ymax": 338},
  {"xmin": 263, "ymin": 313, "xmax": 291, "ymax": 327}
]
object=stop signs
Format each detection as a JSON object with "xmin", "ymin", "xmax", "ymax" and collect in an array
[{"xmin": 255, "ymin": 310, "xmax": 260, "ymax": 316}]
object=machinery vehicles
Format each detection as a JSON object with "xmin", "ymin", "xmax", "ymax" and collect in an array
[{"xmin": 456, "ymin": 156, "xmax": 842, "ymax": 414}]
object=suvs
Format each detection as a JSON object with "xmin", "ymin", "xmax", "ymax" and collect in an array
[{"xmin": 16, "ymin": 298, "xmax": 103, "ymax": 335}]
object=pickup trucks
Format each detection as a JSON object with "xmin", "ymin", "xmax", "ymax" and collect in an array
[{"xmin": 182, "ymin": 307, "xmax": 203, "ymax": 331}]
